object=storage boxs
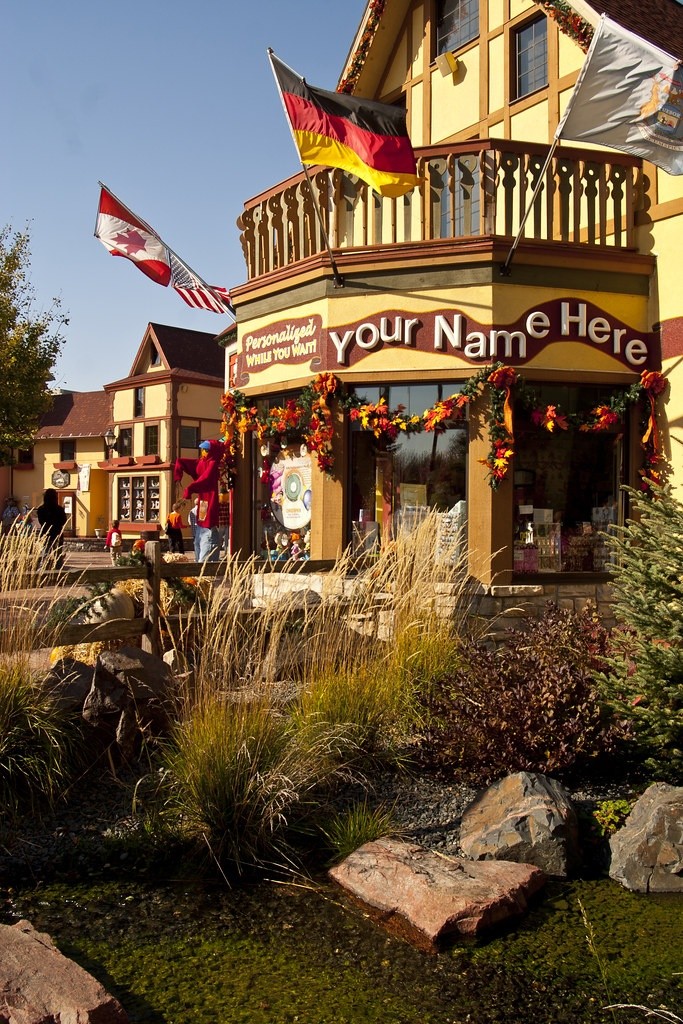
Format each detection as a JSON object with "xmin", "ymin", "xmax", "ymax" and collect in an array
[
  {"xmin": 514, "ymin": 549, "xmax": 538, "ymax": 573},
  {"xmin": 592, "ymin": 507, "xmax": 616, "ymax": 570},
  {"xmin": 533, "ymin": 508, "xmax": 553, "ymax": 523}
]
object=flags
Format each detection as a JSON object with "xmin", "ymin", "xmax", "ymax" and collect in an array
[
  {"xmin": 553, "ymin": 12, "xmax": 683, "ymax": 176},
  {"xmin": 92, "ymin": 185, "xmax": 232, "ymax": 316},
  {"xmin": 266, "ymin": 47, "xmax": 429, "ymax": 198}
]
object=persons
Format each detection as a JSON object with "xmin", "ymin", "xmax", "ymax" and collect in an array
[
  {"xmin": 164, "ymin": 502, "xmax": 186, "ymax": 554},
  {"xmin": 188, "ymin": 497, "xmax": 198, "ymax": 551},
  {"xmin": 174, "ymin": 439, "xmax": 225, "ymax": 562},
  {"xmin": 36, "ymin": 487, "xmax": 67, "ymax": 570},
  {"xmin": 104, "ymin": 519, "xmax": 123, "ymax": 567},
  {"xmin": 2, "ymin": 495, "xmax": 34, "ymax": 555}
]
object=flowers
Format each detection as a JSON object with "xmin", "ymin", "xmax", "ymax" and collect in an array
[{"xmin": 214, "ymin": 362, "xmax": 670, "ymax": 504}]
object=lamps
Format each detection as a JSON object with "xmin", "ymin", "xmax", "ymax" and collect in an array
[{"xmin": 101, "ymin": 428, "xmax": 117, "ymax": 451}]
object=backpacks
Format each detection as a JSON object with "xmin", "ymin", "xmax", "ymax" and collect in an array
[{"xmin": 111, "ymin": 532, "xmax": 121, "ymax": 547}]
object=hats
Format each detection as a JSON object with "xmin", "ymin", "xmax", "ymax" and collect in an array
[{"xmin": 199, "ymin": 441, "xmax": 210, "ymax": 450}]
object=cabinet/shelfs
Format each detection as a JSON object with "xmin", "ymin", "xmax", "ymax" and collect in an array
[{"xmin": 527, "ymin": 522, "xmax": 561, "ymax": 573}]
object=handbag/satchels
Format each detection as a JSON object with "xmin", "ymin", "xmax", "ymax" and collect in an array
[{"xmin": 165, "ymin": 515, "xmax": 170, "ymax": 535}]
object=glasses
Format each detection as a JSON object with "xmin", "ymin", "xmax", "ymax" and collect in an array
[{"xmin": 201, "ymin": 448, "xmax": 208, "ymax": 452}]
